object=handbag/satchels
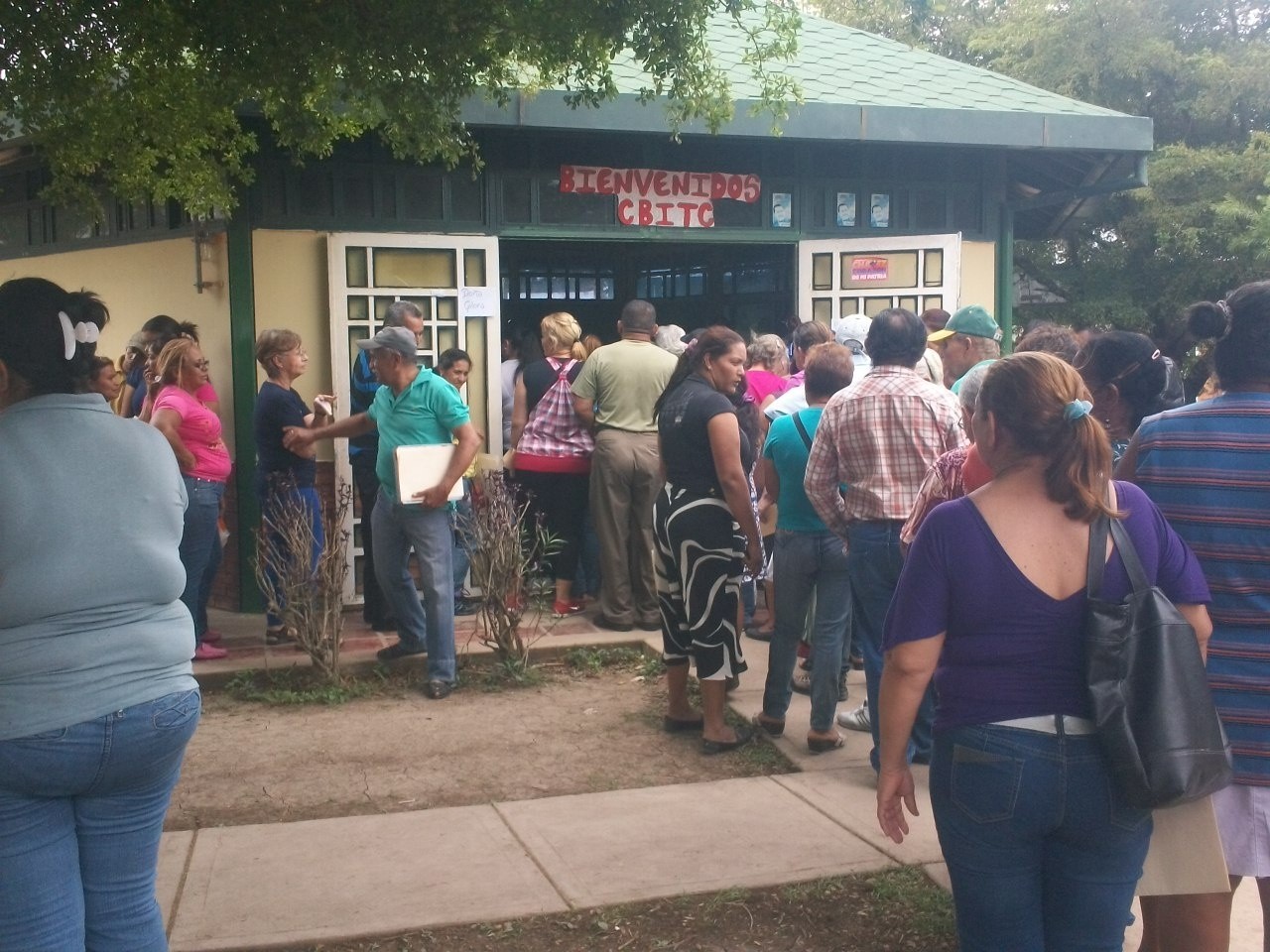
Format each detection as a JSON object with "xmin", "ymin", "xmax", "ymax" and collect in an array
[{"xmin": 1075, "ymin": 504, "xmax": 1237, "ymax": 809}]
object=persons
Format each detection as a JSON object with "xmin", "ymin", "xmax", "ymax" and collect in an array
[
  {"xmin": 85, "ymin": 316, "xmax": 220, "ymax": 438},
  {"xmin": 746, "ymin": 342, "xmax": 853, "ymax": 753},
  {"xmin": 147, "ymin": 337, "xmax": 233, "ymax": 659},
  {"xmin": 500, "ymin": 314, "xmax": 705, "ymax": 601},
  {"xmin": 253, "ymin": 328, "xmax": 337, "ymax": 642},
  {"xmin": 900, "ymin": 306, "xmax": 1188, "ymax": 565},
  {"xmin": 431, "ymin": 345, "xmax": 482, "ymax": 617},
  {"xmin": 803, "ymin": 309, "xmax": 971, "ymax": 771},
  {"xmin": 282, "ymin": 326, "xmax": 482, "ymax": 699},
  {"xmin": 503, "ymin": 313, "xmax": 598, "ymax": 617},
  {"xmin": 570, "ymin": 299, "xmax": 682, "ymax": 631},
  {"xmin": 1114, "ymin": 280, "xmax": 1268, "ymax": 951},
  {"xmin": 348, "ymin": 299, "xmax": 425, "ymax": 630},
  {"xmin": 1, "ymin": 277, "xmax": 205, "ymax": 952},
  {"xmin": 877, "ymin": 353, "xmax": 1215, "ymax": 951},
  {"xmin": 727, "ymin": 308, "xmax": 870, "ymax": 702},
  {"xmin": 650, "ymin": 326, "xmax": 765, "ymax": 757}
]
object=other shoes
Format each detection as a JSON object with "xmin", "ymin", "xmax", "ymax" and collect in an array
[
  {"xmin": 192, "ymin": 643, "xmax": 228, "ymax": 659},
  {"xmin": 198, "ymin": 629, "xmax": 220, "ymax": 641},
  {"xmin": 378, "ymin": 643, "xmax": 424, "ymax": 657},
  {"xmin": 851, "ymin": 660, "xmax": 864, "ymax": 670},
  {"xmin": 423, "ymin": 680, "xmax": 451, "ymax": 700},
  {"xmin": 700, "ymin": 725, "xmax": 752, "ymax": 754},
  {"xmin": 634, "ymin": 621, "xmax": 662, "ymax": 631},
  {"xmin": 662, "ymin": 712, "xmax": 703, "ymax": 733},
  {"xmin": 593, "ymin": 612, "xmax": 632, "ymax": 632},
  {"xmin": 745, "ymin": 626, "xmax": 774, "ymax": 639}
]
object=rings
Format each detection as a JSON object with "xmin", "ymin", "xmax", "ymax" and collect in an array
[{"xmin": 290, "ymin": 429, "xmax": 293, "ymax": 437}]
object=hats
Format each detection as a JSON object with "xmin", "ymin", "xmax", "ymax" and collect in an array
[
  {"xmin": 1072, "ymin": 329, "xmax": 1158, "ymax": 398},
  {"xmin": 833, "ymin": 314, "xmax": 872, "ymax": 354},
  {"xmin": 925, "ymin": 304, "xmax": 1002, "ymax": 344},
  {"xmin": 125, "ymin": 332, "xmax": 148, "ymax": 356}
]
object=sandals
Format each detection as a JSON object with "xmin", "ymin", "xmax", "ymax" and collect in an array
[
  {"xmin": 321, "ymin": 634, "xmax": 344, "ymax": 649},
  {"xmin": 267, "ymin": 625, "xmax": 304, "ymax": 645}
]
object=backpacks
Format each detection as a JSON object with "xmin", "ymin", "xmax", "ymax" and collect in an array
[{"xmin": 513, "ymin": 355, "xmax": 596, "ymax": 476}]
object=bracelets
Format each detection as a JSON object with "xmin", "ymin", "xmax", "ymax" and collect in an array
[{"xmin": 314, "ymin": 409, "xmax": 327, "ymax": 418}]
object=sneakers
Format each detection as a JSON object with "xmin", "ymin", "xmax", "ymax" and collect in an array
[
  {"xmin": 792, "ymin": 664, "xmax": 848, "ymax": 701},
  {"xmin": 553, "ymin": 599, "xmax": 586, "ymax": 618},
  {"xmin": 838, "ymin": 697, "xmax": 872, "ymax": 732},
  {"xmin": 451, "ymin": 592, "xmax": 480, "ymax": 616}
]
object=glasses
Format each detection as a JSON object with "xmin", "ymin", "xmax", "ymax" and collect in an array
[{"xmin": 181, "ymin": 359, "xmax": 209, "ymax": 368}]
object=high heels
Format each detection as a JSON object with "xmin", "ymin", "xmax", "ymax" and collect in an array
[
  {"xmin": 752, "ymin": 711, "xmax": 785, "ymax": 738},
  {"xmin": 806, "ymin": 731, "xmax": 847, "ymax": 754}
]
